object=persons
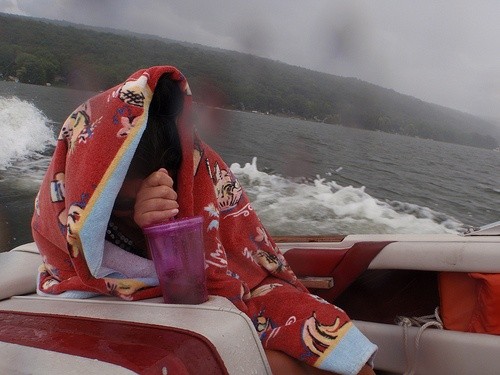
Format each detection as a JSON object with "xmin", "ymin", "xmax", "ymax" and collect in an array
[{"xmin": 31, "ymin": 64, "xmax": 378, "ymax": 375}]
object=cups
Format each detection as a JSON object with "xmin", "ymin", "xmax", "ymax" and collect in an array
[{"xmin": 142, "ymin": 214, "xmax": 208, "ymax": 306}]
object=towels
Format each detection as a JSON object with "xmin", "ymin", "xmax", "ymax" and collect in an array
[{"xmin": 33, "ymin": 63, "xmax": 380, "ymax": 375}]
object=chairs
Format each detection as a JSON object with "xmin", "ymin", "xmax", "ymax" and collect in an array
[{"xmin": 0, "ymin": 233, "xmax": 355, "ymax": 375}]
